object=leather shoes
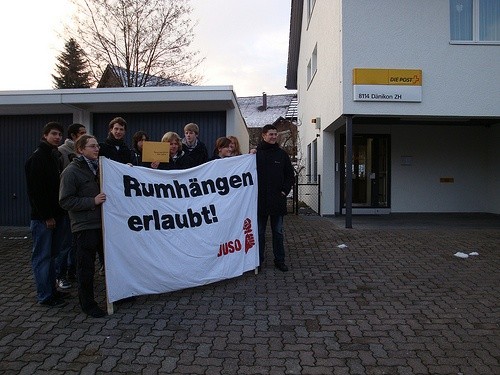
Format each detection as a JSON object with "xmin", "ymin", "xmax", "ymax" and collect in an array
[{"xmin": 274, "ymin": 258, "xmax": 288, "ymax": 272}]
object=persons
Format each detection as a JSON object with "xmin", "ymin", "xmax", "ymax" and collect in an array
[
  {"xmin": 54, "ymin": 124, "xmax": 87, "ymax": 288},
  {"xmin": 130, "ymin": 131, "xmax": 150, "ymax": 167},
  {"xmin": 59, "ymin": 134, "xmax": 133, "ymax": 317},
  {"xmin": 228, "ymin": 136, "xmax": 242, "ymax": 156},
  {"xmin": 214, "ymin": 137, "xmax": 233, "ymax": 159},
  {"xmin": 144, "ymin": 131, "xmax": 191, "ymax": 170},
  {"xmin": 98, "ymin": 117, "xmax": 130, "ymax": 276},
  {"xmin": 250, "ymin": 125, "xmax": 294, "ymax": 272},
  {"xmin": 25, "ymin": 122, "xmax": 64, "ymax": 306},
  {"xmin": 181, "ymin": 123, "xmax": 208, "ymax": 166}
]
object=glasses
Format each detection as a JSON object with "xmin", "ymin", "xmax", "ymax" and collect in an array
[{"xmin": 84, "ymin": 145, "xmax": 101, "ymax": 149}]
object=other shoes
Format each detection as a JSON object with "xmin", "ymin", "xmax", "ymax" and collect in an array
[
  {"xmin": 53, "ymin": 291, "xmax": 70, "ymax": 297},
  {"xmin": 82, "ymin": 304, "xmax": 105, "ymax": 317},
  {"xmin": 39, "ymin": 298, "xmax": 67, "ymax": 307},
  {"xmin": 57, "ymin": 279, "xmax": 73, "ymax": 289}
]
